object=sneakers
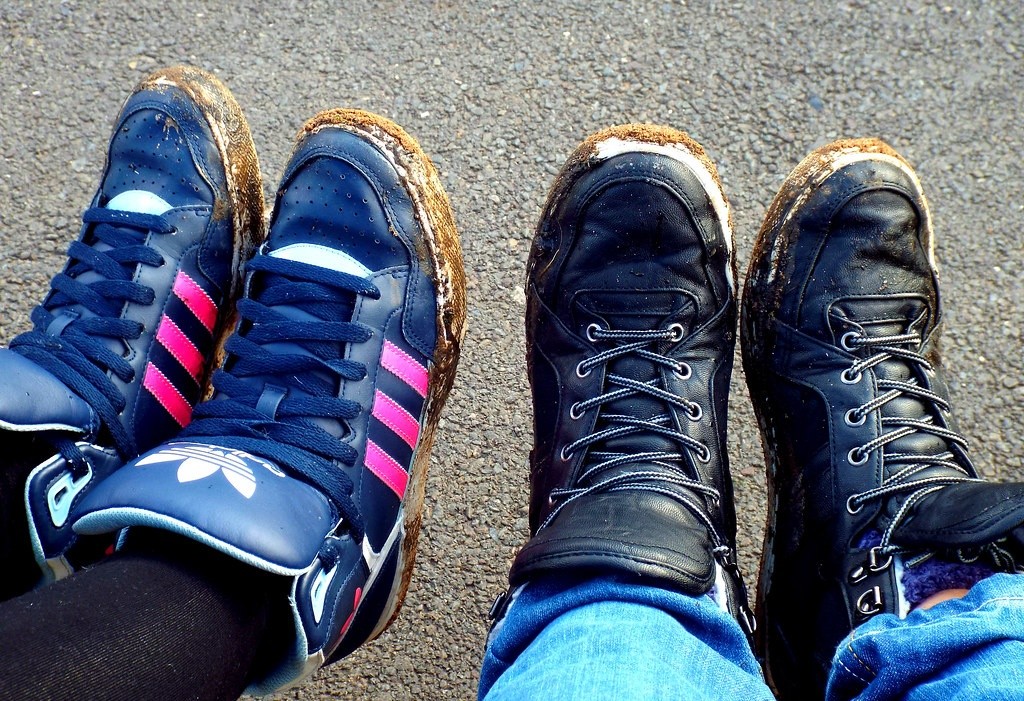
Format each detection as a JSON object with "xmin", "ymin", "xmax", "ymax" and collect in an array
[
  {"xmin": 0, "ymin": 65, "xmax": 264, "ymax": 583},
  {"xmin": 69, "ymin": 109, "xmax": 466, "ymax": 696},
  {"xmin": 740, "ymin": 137, "xmax": 1024, "ymax": 701},
  {"xmin": 485, "ymin": 126, "xmax": 766, "ymax": 682}
]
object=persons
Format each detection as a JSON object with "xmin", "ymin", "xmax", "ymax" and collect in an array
[
  {"xmin": 0, "ymin": 61, "xmax": 467, "ymax": 701},
  {"xmin": 474, "ymin": 122, "xmax": 1024, "ymax": 701}
]
